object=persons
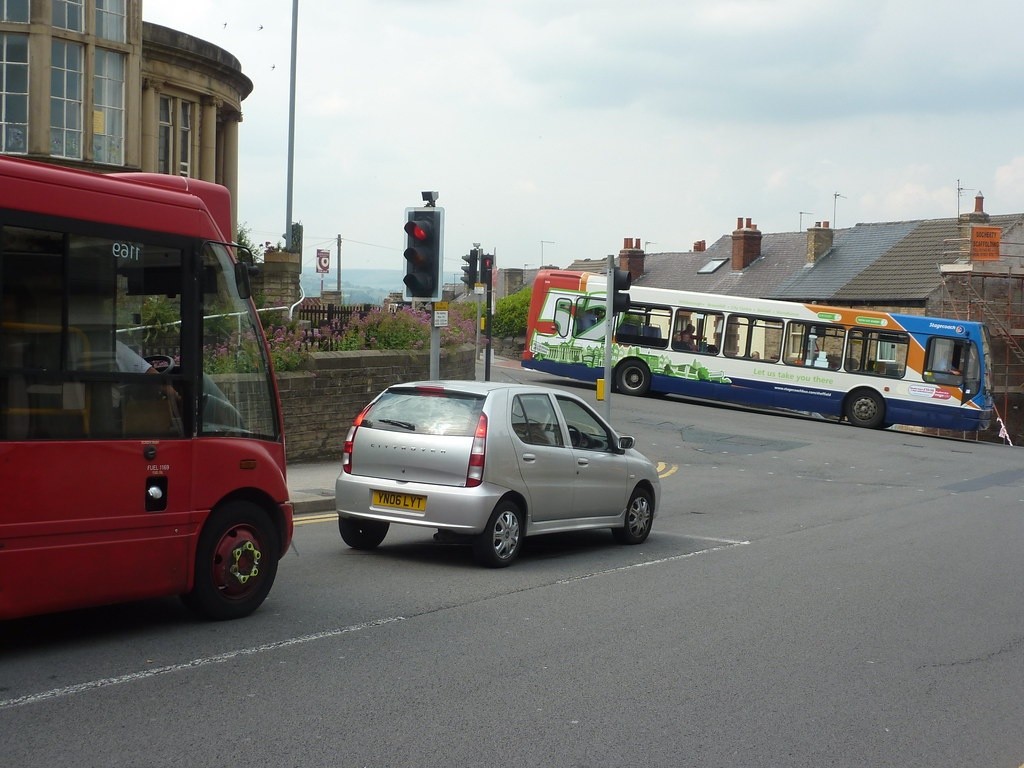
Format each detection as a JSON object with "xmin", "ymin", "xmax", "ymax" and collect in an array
[
  {"xmin": 749, "ymin": 351, "xmax": 759, "ymax": 359},
  {"xmin": 578, "ymin": 307, "xmax": 604, "ymax": 332},
  {"xmin": 672, "ymin": 324, "xmax": 698, "ymax": 352},
  {"xmin": 42, "ymin": 306, "xmax": 179, "ymax": 434},
  {"xmin": 938, "ymin": 351, "xmax": 963, "ymax": 375}
]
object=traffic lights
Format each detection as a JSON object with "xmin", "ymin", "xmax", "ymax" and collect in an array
[
  {"xmin": 460, "ymin": 249, "xmax": 478, "ymax": 290},
  {"xmin": 401, "ymin": 206, "xmax": 445, "ymax": 302},
  {"xmin": 480, "ymin": 255, "xmax": 493, "ymax": 292}
]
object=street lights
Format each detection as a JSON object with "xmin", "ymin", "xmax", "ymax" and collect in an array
[{"xmin": 613, "ymin": 266, "xmax": 632, "ymax": 317}]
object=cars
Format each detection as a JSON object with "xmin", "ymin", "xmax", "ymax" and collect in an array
[{"xmin": 336, "ymin": 380, "xmax": 662, "ymax": 568}]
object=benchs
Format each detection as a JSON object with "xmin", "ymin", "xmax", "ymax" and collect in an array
[{"xmin": 618, "ymin": 321, "xmax": 662, "ymax": 338}]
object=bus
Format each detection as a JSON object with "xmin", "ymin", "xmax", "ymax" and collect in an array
[
  {"xmin": 0, "ymin": 153, "xmax": 292, "ymax": 615},
  {"xmin": 522, "ymin": 269, "xmax": 994, "ymax": 431}
]
